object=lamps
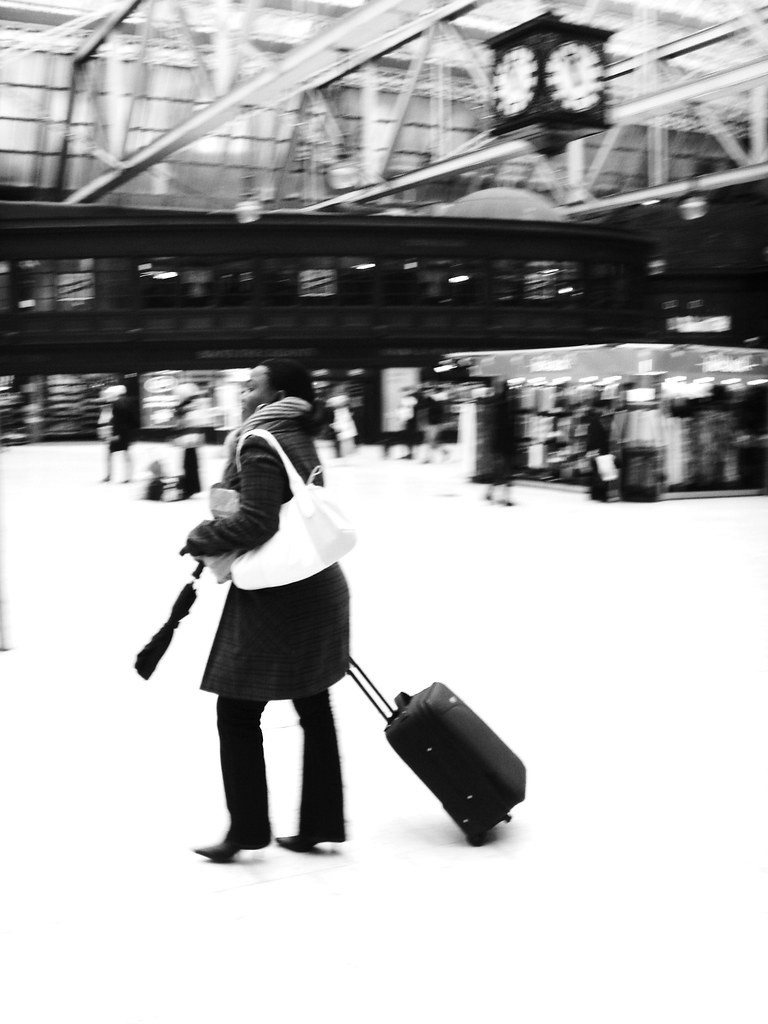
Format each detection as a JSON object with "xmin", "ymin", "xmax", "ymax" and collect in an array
[
  {"xmin": 679, "ymin": 189, "xmax": 707, "ymax": 219},
  {"xmin": 232, "ymin": 171, "xmax": 261, "ymax": 222}
]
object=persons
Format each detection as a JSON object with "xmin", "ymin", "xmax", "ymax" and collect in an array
[
  {"xmin": 173, "ymin": 383, "xmax": 212, "ymax": 501},
  {"xmin": 324, "ymin": 395, "xmax": 358, "ymax": 458},
  {"xmin": 398, "ymin": 385, "xmax": 446, "ymax": 464},
  {"xmin": 580, "ymin": 408, "xmax": 622, "ymax": 503},
  {"xmin": 473, "ymin": 379, "xmax": 521, "ymax": 506},
  {"xmin": 181, "ymin": 355, "xmax": 349, "ymax": 862},
  {"xmin": 101, "ymin": 384, "xmax": 130, "ymax": 484}
]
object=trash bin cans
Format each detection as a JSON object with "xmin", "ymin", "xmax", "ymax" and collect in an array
[{"xmin": 623, "ymin": 447, "xmax": 658, "ymax": 501}]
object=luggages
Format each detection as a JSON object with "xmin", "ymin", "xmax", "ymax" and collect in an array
[{"xmin": 346, "ymin": 654, "xmax": 527, "ymax": 846}]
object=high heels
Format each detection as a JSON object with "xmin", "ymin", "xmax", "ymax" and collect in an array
[
  {"xmin": 194, "ymin": 840, "xmax": 266, "ymax": 861},
  {"xmin": 275, "ymin": 834, "xmax": 339, "ymax": 854}
]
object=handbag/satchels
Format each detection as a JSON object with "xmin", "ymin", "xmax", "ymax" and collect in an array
[
  {"xmin": 209, "ymin": 428, "xmax": 361, "ymax": 587},
  {"xmin": 595, "ymin": 453, "xmax": 620, "ymax": 482}
]
